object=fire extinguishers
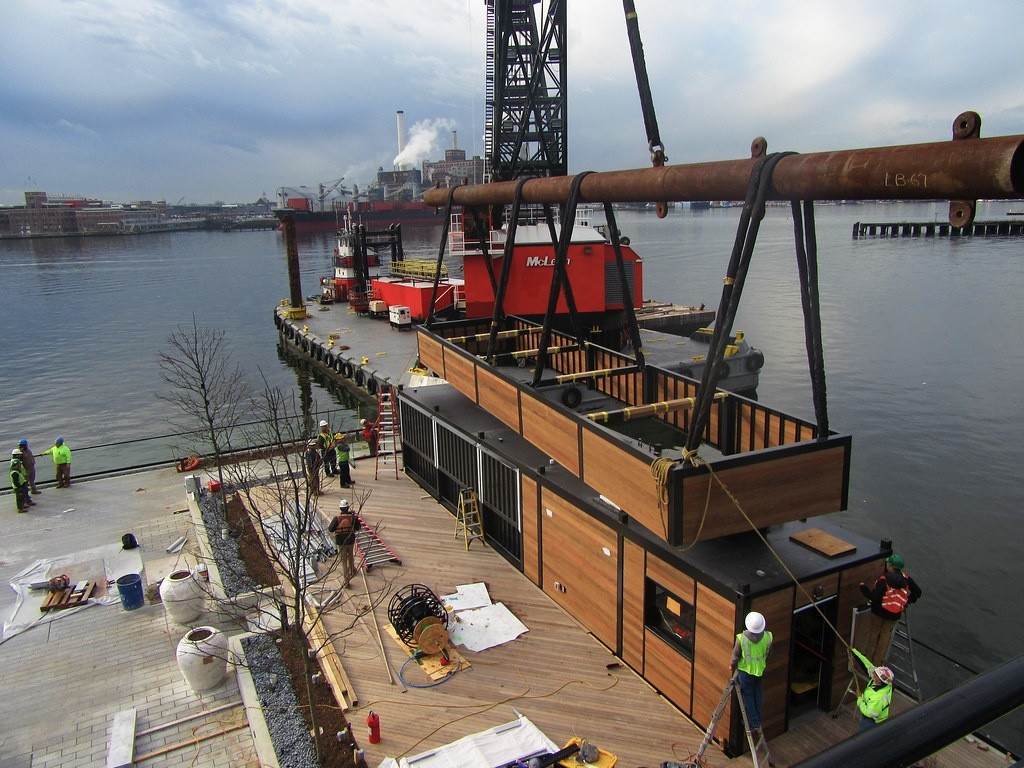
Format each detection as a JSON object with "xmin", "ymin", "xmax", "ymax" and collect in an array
[{"xmin": 367, "ymin": 711, "xmax": 380, "ymax": 744}]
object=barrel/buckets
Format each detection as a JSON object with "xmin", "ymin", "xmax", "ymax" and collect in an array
[{"xmin": 116, "ymin": 573, "xmax": 143, "ymax": 611}]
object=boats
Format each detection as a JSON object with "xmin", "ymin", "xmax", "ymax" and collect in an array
[{"xmin": 273, "ymin": 197, "xmax": 765, "ymax": 412}]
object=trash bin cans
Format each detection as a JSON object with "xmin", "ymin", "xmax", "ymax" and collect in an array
[{"xmin": 117, "ymin": 574, "xmax": 144, "ymax": 612}]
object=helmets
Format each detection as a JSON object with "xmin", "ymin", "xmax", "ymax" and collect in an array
[
  {"xmin": 875, "ymin": 667, "xmax": 894, "ymax": 683},
  {"xmin": 55, "ymin": 437, "xmax": 64, "ymax": 443},
  {"xmin": 885, "ymin": 554, "xmax": 905, "ymax": 569},
  {"xmin": 11, "ymin": 448, "xmax": 22, "ymax": 454},
  {"xmin": 339, "ymin": 499, "xmax": 349, "ymax": 507},
  {"xmin": 360, "ymin": 419, "xmax": 366, "ymax": 426},
  {"xmin": 745, "ymin": 612, "xmax": 766, "ymax": 634},
  {"xmin": 320, "ymin": 420, "xmax": 328, "ymax": 427},
  {"xmin": 12, "ymin": 460, "xmax": 20, "ymax": 466},
  {"xmin": 18, "ymin": 439, "xmax": 28, "ymax": 446},
  {"xmin": 308, "ymin": 439, "xmax": 316, "ymax": 445},
  {"xmin": 334, "ymin": 432, "xmax": 345, "ymax": 439}
]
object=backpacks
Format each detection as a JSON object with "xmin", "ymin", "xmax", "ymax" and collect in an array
[{"xmin": 122, "ymin": 533, "xmax": 137, "ymax": 550}]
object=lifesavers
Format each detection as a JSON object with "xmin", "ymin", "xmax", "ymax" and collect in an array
[
  {"xmin": 274, "ymin": 305, "xmax": 388, "ymax": 403},
  {"xmin": 562, "ymin": 388, "xmax": 582, "ymax": 408},
  {"xmin": 178, "ymin": 455, "xmax": 199, "ymax": 470}
]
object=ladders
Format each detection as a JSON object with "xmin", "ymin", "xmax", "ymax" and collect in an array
[
  {"xmin": 832, "ymin": 605, "xmax": 923, "ymax": 737},
  {"xmin": 350, "ymin": 510, "xmax": 402, "ymax": 573},
  {"xmin": 694, "ymin": 669, "xmax": 775, "ymax": 768},
  {"xmin": 453, "ymin": 487, "xmax": 486, "ymax": 552},
  {"xmin": 375, "ymin": 383, "xmax": 404, "ymax": 480}
]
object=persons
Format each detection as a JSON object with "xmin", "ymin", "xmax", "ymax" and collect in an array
[
  {"xmin": 317, "ymin": 420, "xmax": 340, "ymax": 477},
  {"xmin": 849, "ymin": 647, "xmax": 894, "ymax": 732},
  {"xmin": 334, "ymin": 433, "xmax": 356, "ymax": 488},
  {"xmin": 360, "ymin": 419, "xmax": 380, "ymax": 453},
  {"xmin": 40, "ymin": 436, "xmax": 72, "ymax": 489},
  {"xmin": 729, "ymin": 612, "xmax": 774, "ymax": 728},
  {"xmin": 10, "ymin": 449, "xmax": 36, "ymax": 513},
  {"xmin": 18, "ymin": 439, "xmax": 41, "ymax": 497},
  {"xmin": 859, "ymin": 553, "xmax": 921, "ymax": 666},
  {"xmin": 305, "ymin": 439, "xmax": 325, "ymax": 496},
  {"xmin": 328, "ymin": 500, "xmax": 361, "ymax": 588}
]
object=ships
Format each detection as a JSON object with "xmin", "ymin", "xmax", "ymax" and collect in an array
[{"xmin": 271, "ymin": 197, "xmax": 452, "ymax": 234}]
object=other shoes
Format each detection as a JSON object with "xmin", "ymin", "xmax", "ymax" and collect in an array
[
  {"xmin": 18, "ymin": 509, "xmax": 28, "ymax": 513},
  {"xmin": 346, "ymin": 481, "xmax": 355, "ymax": 484},
  {"xmin": 31, "ymin": 490, "xmax": 41, "ymax": 494},
  {"xmin": 22, "ymin": 505, "xmax": 29, "ymax": 509},
  {"xmin": 341, "ymin": 485, "xmax": 350, "ymax": 488},
  {"xmin": 63, "ymin": 484, "xmax": 70, "ymax": 487},
  {"xmin": 343, "ymin": 580, "xmax": 350, "ymax": 589},
  {"xmin": 57, "ymin": 484, "xmax": 64, "ymax": 488},
  {"xmin": 742, "ymin": 719, "xmax": 760, "ymax": 728},
  {"xmin": 26, "ymin": 502, "xmax": 36, "ymax": 506}
]
